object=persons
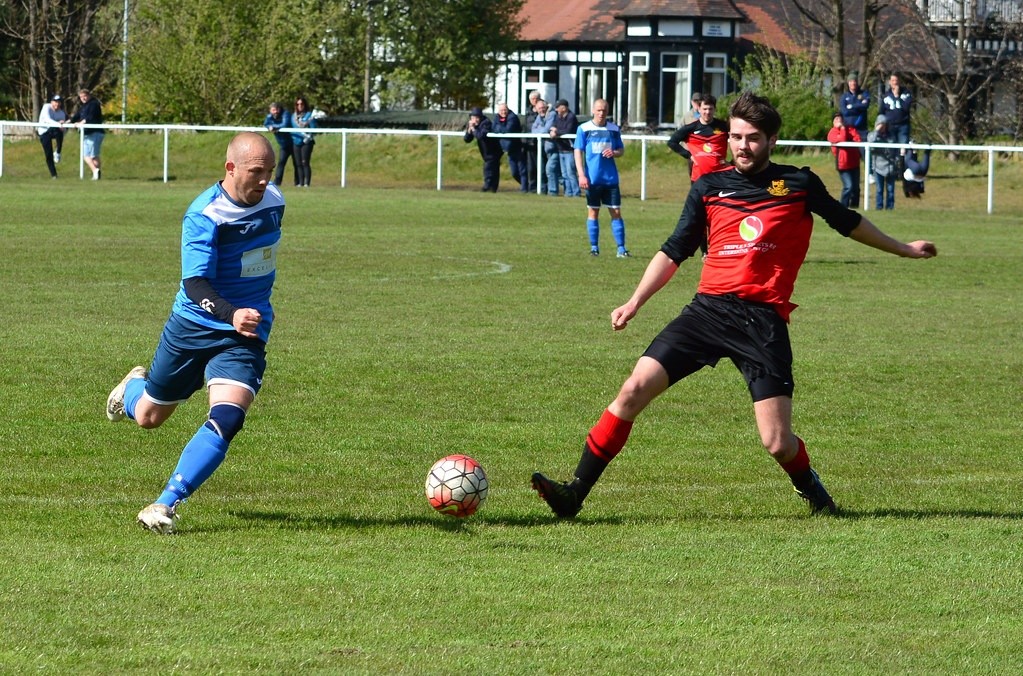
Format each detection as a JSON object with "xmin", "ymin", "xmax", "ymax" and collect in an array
[
  {"xmin": 462, "ymin": 71, "xmax": 931, "ymax": 258},
  {"xmin": 106, "ymin": 133, "xmax": 286, "ymax": 537},
  {"xmin": 38, "ymin": 96, "xmax": 66, "ymax": 181},
  {"xmin": 68, "ymin": 88, "xmax": 105, "ymax": 180},
  {"xmin": 265, "ymin": 97, "xmax": 315, "ymax": 188},
  {"xmin": 528, "ymin": 93, "xmax": 937, "ymax": 522}
]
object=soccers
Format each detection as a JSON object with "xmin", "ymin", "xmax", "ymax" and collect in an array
[{"xmin": 424, "ymin": 454, "xmax": 489, "ymax": 518}]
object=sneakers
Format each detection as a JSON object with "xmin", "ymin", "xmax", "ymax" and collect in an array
[
  {"xmin": 136, "ymin": 500, "xmax": 181, "ymax": 534},
  {"xmin": 53, "ymin": 174, "xmax": 57, "ymax": 179},
  {"xmin": 590, "ymin": 249, "xmax": 599, "ymax": 257},
  {"xmin": 105, "ymin": 366, "xmax": 148, "ymax": 421},
  {"xmin": 53, "ymin": 151, "xmax": 61, "ymax": 163},
  {"xmin": 530, "ymin": 471, "xmax": 583, "ymax": 517},
  {"xmin": 90, "ymin": 168, "xmax": 102, "ymax": 181},
  {"xmin": 616, "ymin": 252, "xmax": 632, "ymax": 259},
  {"xmin": 794, "ymin": 470, "xmax": 836, "ymax": 516}
]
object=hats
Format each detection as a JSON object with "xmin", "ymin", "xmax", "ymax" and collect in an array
[
  {"xmin": 555, "ymin": 99, "xmax": 568, "ymax": 107},
  {"xmin": 692, "ymin": 92, "xmax": 702, "ymax": 100},
  {"xmin": 469, "ymin": 108, "xmax": 482, "ymax": 116},
  {"xmin": 52, "ymin": 95, "xmax": 62, "ymax": 101},
  {"xmin": 847, "ymin": 73, "xmax": 858, "ymax": 82}
]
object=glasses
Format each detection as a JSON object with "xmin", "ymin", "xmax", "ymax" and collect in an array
[{"xmin": 297, "ymin": 103, "xmax": 303, "ymax": 106}]
